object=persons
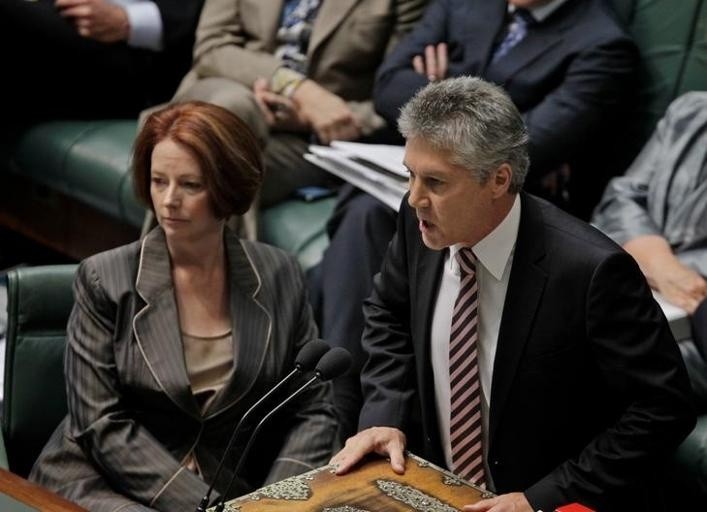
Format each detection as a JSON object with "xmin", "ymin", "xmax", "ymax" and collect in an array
[
  {"xmin": 329, "ymin": 76, "xmax": 699, "ymax": 512},
  {"xmin": 26, "ymin": 101, "xmax": 338, "ymax": 512},
  {"xmin": 0, "ymin": 0, "xmax": 206, "ymax": 164},
  {"xmin": 318, "ymin": 0, "xmax": 637, "ymax": 353},
  {"xmin": 171, "ymin": 0, "xmax": 427, "ymax": 245},
  {"xmin": 588, "ymin": 90, "xmax": 707, "ymax": 413}
]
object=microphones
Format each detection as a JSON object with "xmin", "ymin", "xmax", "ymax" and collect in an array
[
  {"xmin": 194, "ymin": 337, "xmax": 331, "ymax": 512},
  {"xmin": 214, "ymin": 346, "xmax": 354, "ymax": 512}
]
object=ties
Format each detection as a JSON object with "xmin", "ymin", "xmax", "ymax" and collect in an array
[
  {"xmin": 276, "ymin": 1, "xmax": 320, "ymax": 66},
  {"xmin": 449, "ymin": 247, "xmax": 489, "ymax": 495},
  {"xmin": 492, "ymin": 9, "xmax": 530, "ymax": 73}
]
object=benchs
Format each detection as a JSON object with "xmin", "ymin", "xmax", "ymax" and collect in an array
[{"xmin": 12, "ymin": 4, "xmax": 706, "ymax": 275}]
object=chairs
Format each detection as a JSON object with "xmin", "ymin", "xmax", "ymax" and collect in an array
[{"xmin": 6, "ymin": 268, "xmax": 92, "ymax": 471}]
